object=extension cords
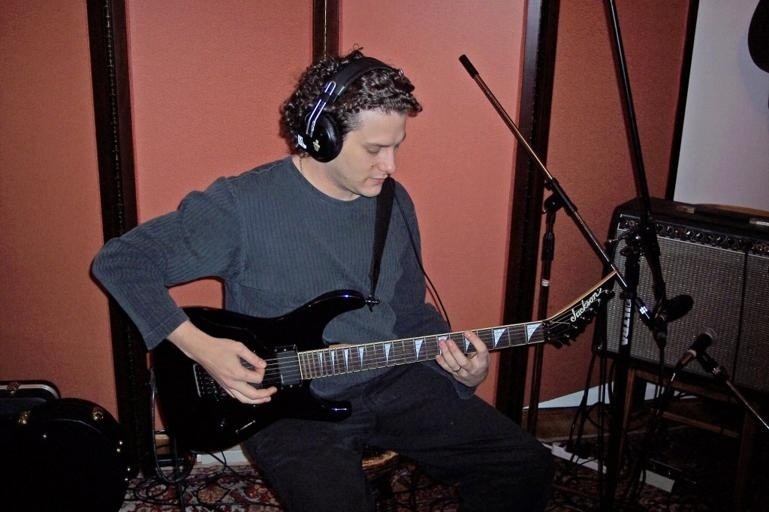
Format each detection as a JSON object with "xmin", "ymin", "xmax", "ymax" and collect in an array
[{"xmin": 551, "ymin": 442, "xmax": 607, "ymax": 475}]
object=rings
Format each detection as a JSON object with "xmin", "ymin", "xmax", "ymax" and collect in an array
[{"xmin": 453, "ymin": 366, "xmax": 461, "ymax": 372}]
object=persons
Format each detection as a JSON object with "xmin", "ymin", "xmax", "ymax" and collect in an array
[{"xmin": 90, "ymin": 42, "xmax": 547, "ymax": 511}]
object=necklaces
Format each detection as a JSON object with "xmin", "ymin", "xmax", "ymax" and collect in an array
[{"xmin": 294, "ymin": 154, "xmax": 309, "ymax": 176}]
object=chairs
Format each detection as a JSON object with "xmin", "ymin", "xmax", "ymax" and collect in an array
[{"xmin": 603, "ymin": 205, "xmax": 769, "ymax": 511}]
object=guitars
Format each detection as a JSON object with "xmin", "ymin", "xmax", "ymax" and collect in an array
[{"xmin": 148, "ymin": 271, "xmax": 615, "ymax": 454}]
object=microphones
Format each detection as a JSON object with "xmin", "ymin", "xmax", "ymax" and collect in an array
[{"xmin": 676, "ymin": 327, "xmax": 718, "ymax": 373}]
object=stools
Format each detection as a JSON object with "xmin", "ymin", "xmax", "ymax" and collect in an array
[{"xmin": 362, "ymin": 447, "xmax": 400, "ymax": 511}]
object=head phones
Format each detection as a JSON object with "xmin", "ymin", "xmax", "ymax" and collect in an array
[{"xmin": 298, "ymin": 57, "xmax": 395, "ymax": 163}]
